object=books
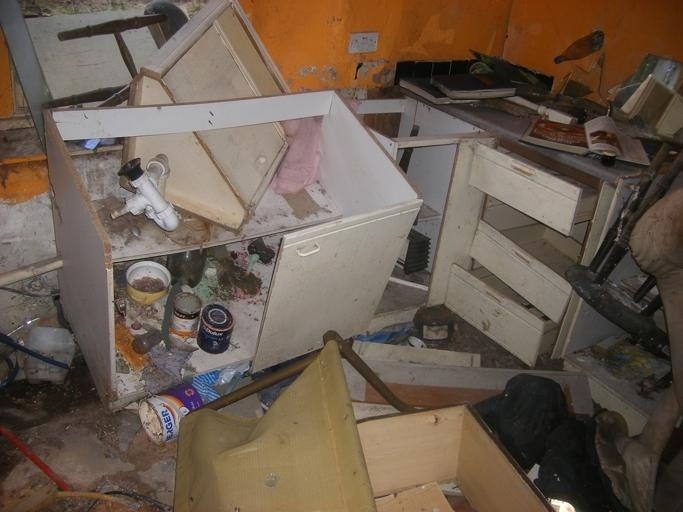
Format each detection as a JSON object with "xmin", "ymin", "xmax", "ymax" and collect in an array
[
  {"xmin": 505, "ymin": 72, "xmax": 683, "ymax": 138},
  {"xmin": 429, "ymin": 72, "xmax": 517, "ymax": 100},
  {"xmin": 517, "ymin": 115, "xmax": 651, "ymax": 167},
  {"xmin": 398, "ymin": 75, "xmax": 481, "ymax": 105}
]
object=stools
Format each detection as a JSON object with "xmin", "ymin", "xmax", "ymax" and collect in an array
[{"xmin": 563, "ymin": 128, "xmax": 681, "ymax": 344}]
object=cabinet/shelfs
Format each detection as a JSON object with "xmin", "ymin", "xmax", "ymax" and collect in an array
[
  {"xmin": 397, "ymin": 76, "xmax": 666, "ymax": 366},
  {"xmin": 41, "ymin": 87, "xmax": 427, "ymax": 415}
]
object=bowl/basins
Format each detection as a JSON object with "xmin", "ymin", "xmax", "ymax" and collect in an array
[{"xmin": 125, "ymin": 261, "xmax": 172, "ymax": 305}]
